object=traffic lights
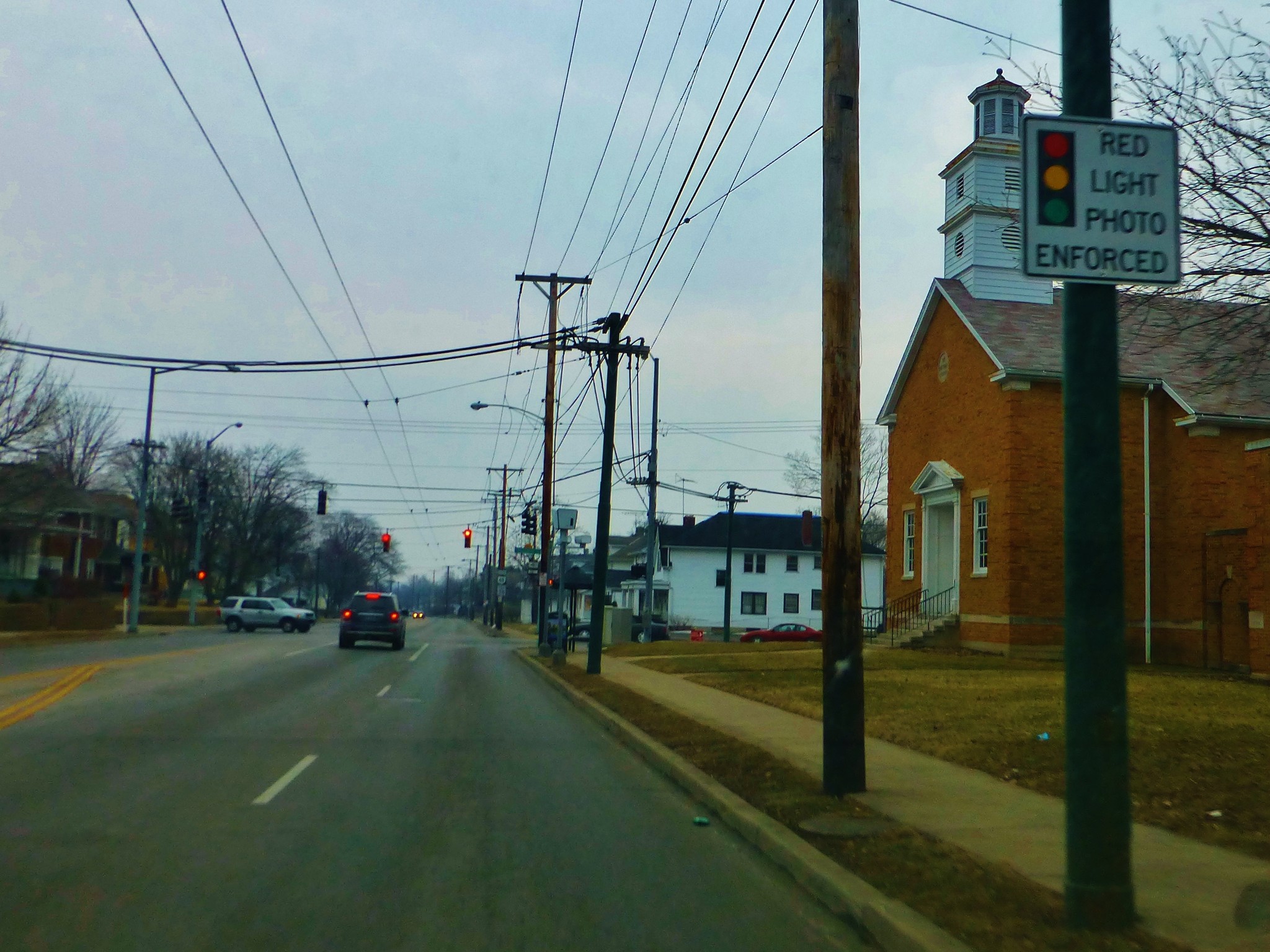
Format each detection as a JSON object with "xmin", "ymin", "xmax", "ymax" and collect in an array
[
  {"xmin": 522, "ymin": 512, "xmax": 530, "ymax": 534},
  {"xmin": 530, "ymin": 514, "xmax": 537, "ymax": 537},
  {"xmin": 548, "ymin": 578, "xmax": 560, "ymax": 590},
  {"xmin": 464, "ymin": 530, "xmax": 471, "ymax": 548},
  {"xmin": 197, "ymin": 570, "xmax": 207, "ymax": 580},
  {"xmin": 383, "ymin": 533, "xmax": 390, "ymax": 553}
]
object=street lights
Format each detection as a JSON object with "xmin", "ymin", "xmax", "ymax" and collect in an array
[
  {"xmin": 470, "ymin": 400, "xmax": 554, "ymax": 650},
  {"xmin": 187, "ymin": 421, "xmax": 244, "ymax": 626},
  {"xmin": 127, "ymin": 361, "xmax": 241, "ymax": 633}
]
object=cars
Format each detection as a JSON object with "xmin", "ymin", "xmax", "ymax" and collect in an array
[
  {"xmin": 548, "ymin": 610, "xmax": 567, "ymax": 627},
  {"xmin": 566, "ymin": 613, "xmax": 671, "ymax": 644},
  {"xmin": 740, "ymin": 623, "xmax": 823, "ymax": 644},
  {"xmin": 413, "ymin": 611, "xmax": 425, "ymax": 619}
]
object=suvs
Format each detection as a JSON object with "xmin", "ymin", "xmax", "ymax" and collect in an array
[
  {"xmin": 340, "ymin": 590, "xmax": 410, "ymax": 651},
  {"xmin": 218, "ymin": 596, "xmax": 316, "ymax": 634},
  {"xmin": 281, "ymin": 594, "xmax": 311, "ymax": 609}
]
